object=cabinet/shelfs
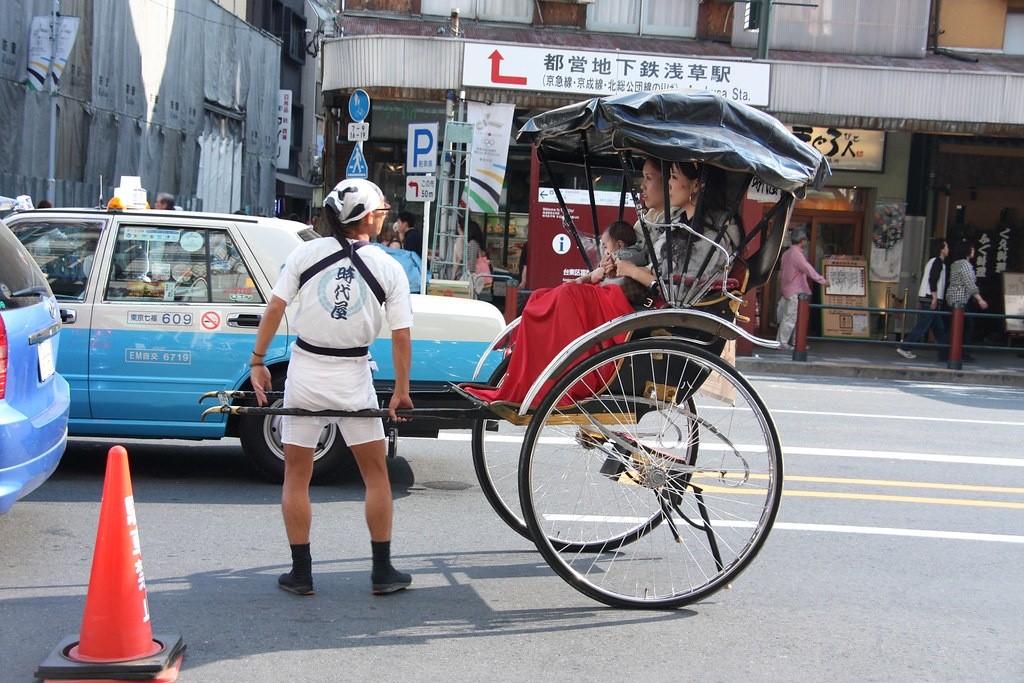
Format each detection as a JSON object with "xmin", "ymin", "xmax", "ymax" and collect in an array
[{"xmin": 466, "ymin": 210, "xmax": 529, "ymax": 276}]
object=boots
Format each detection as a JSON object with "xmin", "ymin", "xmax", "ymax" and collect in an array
[
  {"xmin": 278, "ymin": 542, "xmax": 315, "ymax": 595},
  {"xmin": 372, "ymin": 540, "xmax": 411, "ymax": 593}
]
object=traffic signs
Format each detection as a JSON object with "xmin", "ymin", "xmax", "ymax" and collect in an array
[{"xmin": 405, "ymin": 176, "xmax": 436, "ymax": 201}]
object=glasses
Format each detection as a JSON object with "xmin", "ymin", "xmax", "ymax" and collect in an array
[{"xmin": 375, "ymin": 203, "xmax": 390, "ymax": 213}]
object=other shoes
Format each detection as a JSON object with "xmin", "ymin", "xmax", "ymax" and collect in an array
[
  {"xmin": 778, "ymin": 344, "xmax": 794, "ymax": 350},
  {"xmin": 897, "ymin": 348, "xmax": 916, "ymax": 359}
]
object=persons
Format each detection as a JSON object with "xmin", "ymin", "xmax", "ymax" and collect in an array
[
  {"xmin": 232, "ymin": 207, "xmax": 313, "ymax": 228},
  {"xmin": 397, "ymin": 210, "xmax": 430, "ymax": 270},
  {"xmin": 50, "ymin": 240, "xmax": 97, "ymax": 296},
  {"xmin": 452, "ymin": 215, "xmax": 493, "ymax": 301},
  {"xmin": 896, "ymin": 237, "xmax": 949, "ymax": 359},
  {"xmin": 31, "ymin": 199, "xmax": 74, "ymax": 254},
  {"xmin": 154, "ymin": 192, "xmax": 175, "ymax": 210},
  {"xmin": 937, "ymin": 242, "xmax": 987, "ymax": 362},
  {"xmin": 776, "ymin": 227, "xmax": 831, "ymax": 351},
  {"xmin": 250, "ymin": 179, "xmax": 414, "ymax": 596},
  {"xmin": 507, "ymin": 151, "xmax": 739, "ymax": 399},
  {"xmin": 515, "ymin": 223, "xmax": 529, "ymax": 317}
]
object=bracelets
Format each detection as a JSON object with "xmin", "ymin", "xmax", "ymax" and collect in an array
[
  {"xmin": 648, "ymin": 278, "xmax": 659, "ymax": 288},
  {"xmin": 252, "ymin": 350, "xmax": 266, "ymax": 357},
  {"xmin": 249, "ymin": 363, "xmax": 266, "ymax": 367}
]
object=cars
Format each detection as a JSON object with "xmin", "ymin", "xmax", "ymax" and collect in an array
[
  {"xmin": 0, "ymin": 221, "xmax": 71, "ymax": 512},
  {"xmin": 4, "ymin": 208, "xmax": 509, "ymax": 488}
]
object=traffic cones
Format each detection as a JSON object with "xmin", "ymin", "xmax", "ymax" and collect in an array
[{"xmin": 34, "ymin": 445, "xmax": 187, "ymax": 683}]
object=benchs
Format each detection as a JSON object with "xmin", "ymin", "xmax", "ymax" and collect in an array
[{"xmin": 639, "ymin": 260, "xmax": 749, "ymax": 340}]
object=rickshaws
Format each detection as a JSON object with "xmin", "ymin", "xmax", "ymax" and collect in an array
[{"xmin": 198, "ymin": 90, "xmax": 834, "ymax": 611}]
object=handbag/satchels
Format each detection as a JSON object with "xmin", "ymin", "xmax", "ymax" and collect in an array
[{"xmin": 468, "ymin": 256, "xmax": 493, "ymax": 286}]
